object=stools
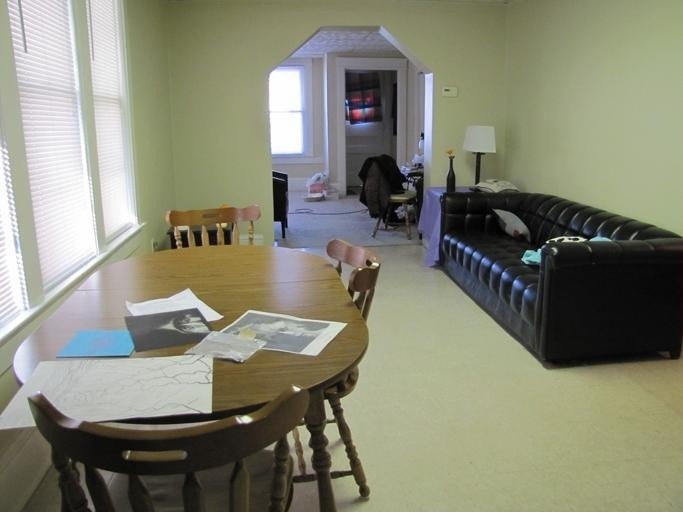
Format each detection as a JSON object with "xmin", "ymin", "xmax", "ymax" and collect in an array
[{"xmin": 368, "ymin": 190, "xmax": 419, "ymax": 241}]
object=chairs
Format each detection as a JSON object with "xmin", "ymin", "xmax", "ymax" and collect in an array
[
  {"xmin": 272, "ymin": 171, "xmax": 291, "ymax": 239},
  {"xmin": 162, "ymin": 203, "xmax": 265, "ymax": 250},
  {"xmin": 289, "ymin": 237, "xmax": 384, "ymax": 501},
  {"xmin": 21, "ymin": 381, "xmax": 310, "ymax": 511}
]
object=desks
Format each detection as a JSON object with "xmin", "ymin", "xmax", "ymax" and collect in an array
[
  {"xmin": 165, "ymin": 219, "xmax": 239, "ymax": 247},
  {"xmin": 400, "ymin": 165, "xmax": 424, "ymax": 188},
  {"xmin": 416, "ymin": 184, "xmax": 483, "ymax": 269},
  {"xmin": 5, "ymin": 242, "xmax": 370, "ymax": 511}
]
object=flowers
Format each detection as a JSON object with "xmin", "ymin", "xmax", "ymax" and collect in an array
[{"xmin": 446, "ymin": 148, "xmax": 454, "ymax": 159}]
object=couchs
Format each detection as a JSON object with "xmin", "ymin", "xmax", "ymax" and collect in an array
[{"xmin": 436, "ymin": 187, "xmax": 682, "ymax": 370}]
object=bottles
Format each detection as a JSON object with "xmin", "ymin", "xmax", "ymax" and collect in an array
[{"xmin": 418, "ymin": 136, "xmax": 425, "ymax": 158}]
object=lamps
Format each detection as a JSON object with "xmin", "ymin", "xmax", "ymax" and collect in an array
[{"xmin": 459, "ymin": 124, "xmax": 500, "ymax": 193}]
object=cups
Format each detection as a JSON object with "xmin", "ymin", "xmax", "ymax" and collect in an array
[{"xmin": 414, "ymin": 162, "xmax": 421, "ymax": 167}]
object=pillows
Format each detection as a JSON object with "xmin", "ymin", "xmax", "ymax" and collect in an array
[
  {"xmin": 472, "ymin": 177, "xmax": 521, "ymax": 197},
  {"xmin": 489, "ymin": 205, "xmax": 535, "ymax": 242},
  {"xmin": 545, "ymin": 233, "xmax": 587, "ymax": 245}
]
